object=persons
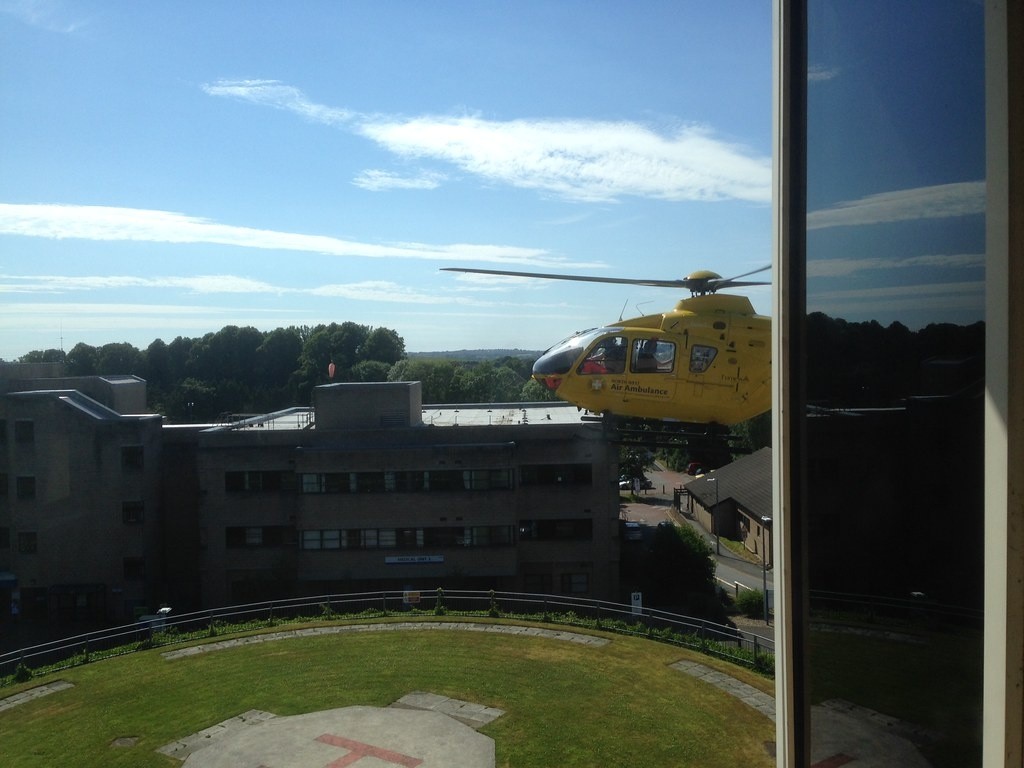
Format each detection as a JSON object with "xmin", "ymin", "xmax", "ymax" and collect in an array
[
  {"xmin": 638, "ymin": 340, "xmax": 673, "ymax": 372},
  {"xmin": 584, "ymin": 336, "xmax": 623, "ymax": 373}
]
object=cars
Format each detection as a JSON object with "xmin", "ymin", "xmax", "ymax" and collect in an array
[
  {"xmin": 625, "ymin": 522, "xmax": 643, "ymax": 541},
  {"xmin": 658, "ymin": 522, "xmax": 674, "ymax": 529},
  {"xmin": 620, "ymin": 475, "xmax": 652, "ymax": 489},
  {"xmin": 686, "ymin": 462, "xmax": 708, "ymax": 476}
]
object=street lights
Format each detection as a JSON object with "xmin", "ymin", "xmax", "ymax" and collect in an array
[
  {"xmin": 762, "ymin": 515, "xmax": 771, "ymax": 624},
  {"xmin": 706, "ymin": 476, "xmax": 719, "ymax": 555}
]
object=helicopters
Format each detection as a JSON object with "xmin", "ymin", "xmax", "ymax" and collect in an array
[{"xmin": 441, "ymin": 265, "xmax": 773, "ymax": 470}]
